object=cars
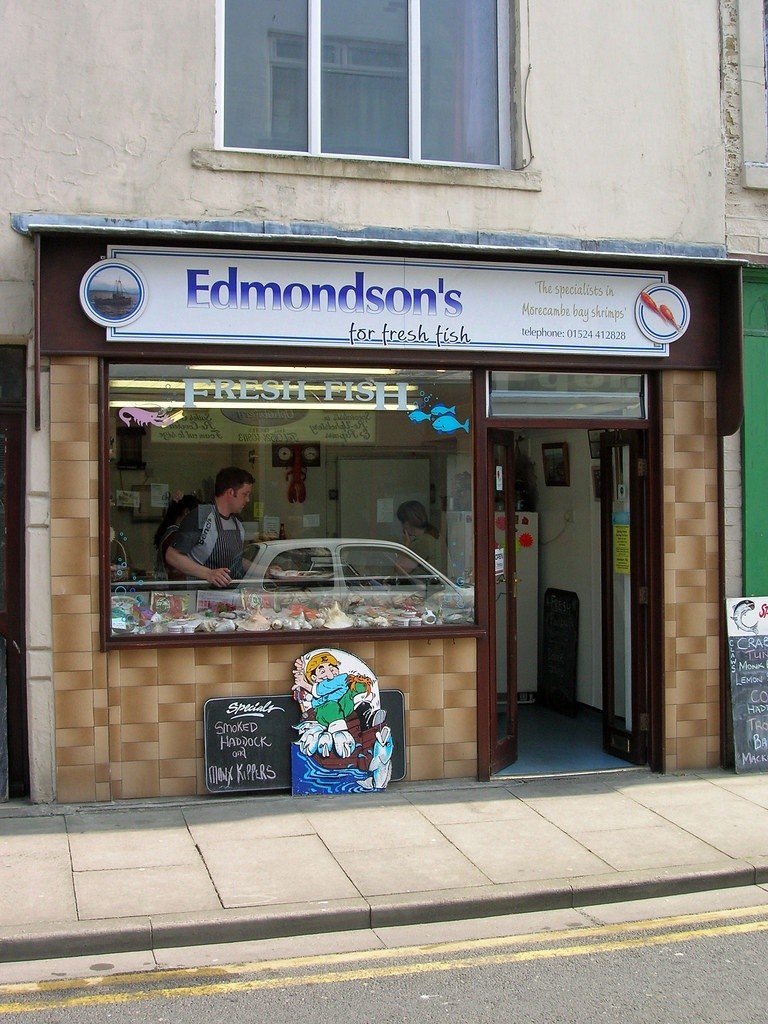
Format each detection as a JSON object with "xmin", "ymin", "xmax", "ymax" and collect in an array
[{"xmin": 236, "ymin": 539, "xmax": 474, "ymax": 610}]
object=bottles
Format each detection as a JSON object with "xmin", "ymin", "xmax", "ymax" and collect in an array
[{"xmin": 279, "ymin": 523, "xmax": 285, "ymax": 539}]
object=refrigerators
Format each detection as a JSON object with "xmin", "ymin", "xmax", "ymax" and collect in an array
[{"xmin": 446, "ymin": 510, "xmax": 538, "ymax": 703}]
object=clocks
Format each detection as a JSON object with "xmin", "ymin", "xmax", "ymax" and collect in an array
[{"xmin": 272, "ymin": 442, "xmax": 321, "ymax": 467}]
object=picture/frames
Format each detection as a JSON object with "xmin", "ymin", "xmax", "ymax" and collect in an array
[
  {"xmin": 592, "ymin": 466, "xmax": 601, "ymax": 501},
  {"xmin": 587, "ymin": 428, "xmax": 608, "ymax": 459},
  {"xmin": 540, "ymin": 442, "xmax": 571, "ymax": 488}
]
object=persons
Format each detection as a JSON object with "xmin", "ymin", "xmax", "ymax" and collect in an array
[
  {"xmin": 394, "ymin": 501, "xmax": 441, "ymax": 584},
  {"xmin": 165, "ymin": 467, "xmax": 283, "ymax": 591},
  {"xmin": 152, "ymin": 491, "xmax": 203, "ymax": 592}
]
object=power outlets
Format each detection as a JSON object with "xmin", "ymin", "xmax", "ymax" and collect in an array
[{"xmin": 566, "ymin": 511, "xmax": 575, "ymax": 523}]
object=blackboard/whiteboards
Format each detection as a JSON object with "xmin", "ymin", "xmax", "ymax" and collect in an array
[
  {"xmin": 203, "ymin": 689, "xmax": 407, "ymax": 792},
  {"xmin": 730, "ymin": 635, "xmax": 768, "ymax": 774},
  {"xmin": 538, "ymin": 589, "xmax": 579, "ymax": 720}
]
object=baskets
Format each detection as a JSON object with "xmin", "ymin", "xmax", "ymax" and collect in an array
[{"xmin": 110, "ymin": 539, "xmax": 130, "ymax": 582}]
